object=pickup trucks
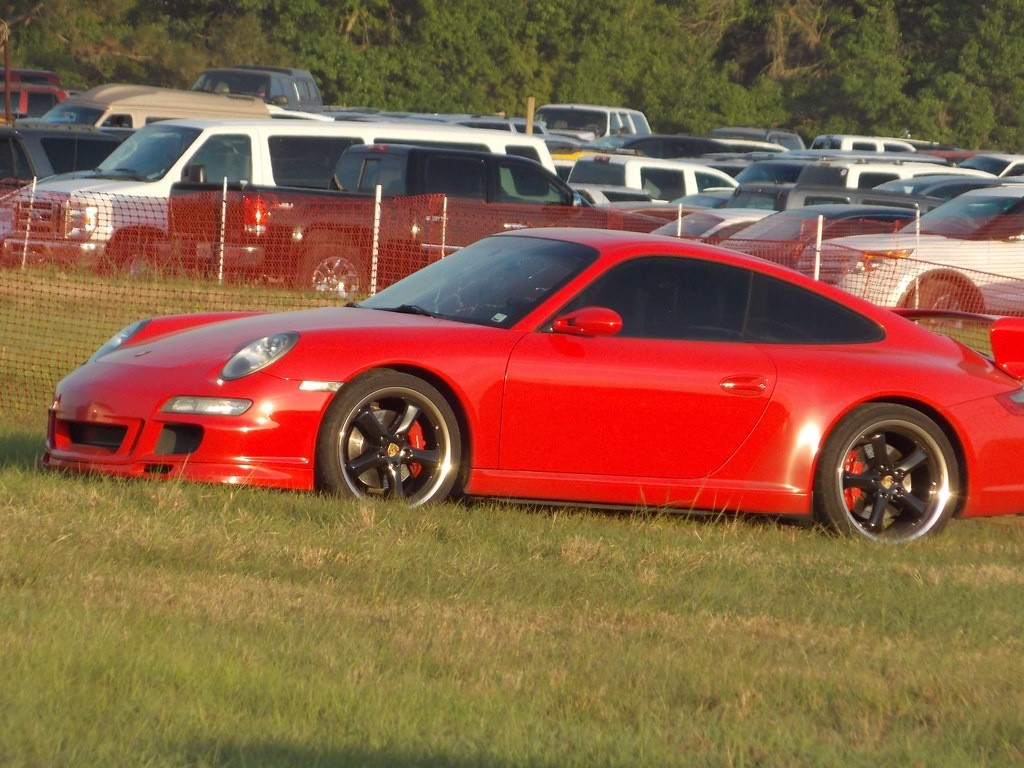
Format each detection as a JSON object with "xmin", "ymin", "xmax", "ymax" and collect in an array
[{"xmin": 165, "ymin": 143, "xmax": 677, "ymax": 299}]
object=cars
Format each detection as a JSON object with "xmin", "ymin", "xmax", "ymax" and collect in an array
[
  {"xmin": 795, "ymin": 186, "xmax": 1024, "ymax": 326},
  {"xmin": 0, "ymin": 58, "xmax": 1024, "ymax": 279},
  {"xmin": 718, "ymin": 203, "xmax": 926, "ymax": 267}
]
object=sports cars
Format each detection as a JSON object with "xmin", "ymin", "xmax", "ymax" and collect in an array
[{"xmin": 43, "ymin": 228, "xmax": 1024, "ymax": 548}]
